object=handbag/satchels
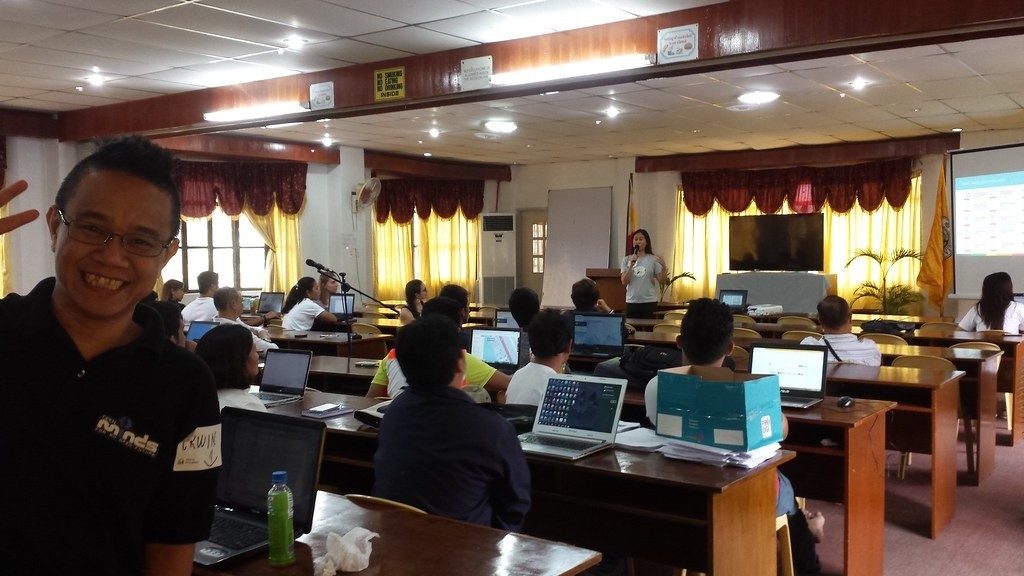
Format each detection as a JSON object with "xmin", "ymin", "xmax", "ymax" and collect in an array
[{"xmin": 859, "ymin": 318, "xmax": 916, "ymax": 340}]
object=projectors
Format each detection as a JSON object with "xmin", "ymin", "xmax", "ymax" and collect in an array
[{"xmin": 747, "ymin": 303, "xmax": 783, "ymax": 316}]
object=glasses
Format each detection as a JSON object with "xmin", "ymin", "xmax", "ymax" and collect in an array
[{"xmin": 57, "ymin": 207, "xmax": 174, "ymax": 258}]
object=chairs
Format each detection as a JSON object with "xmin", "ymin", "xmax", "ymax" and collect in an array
[
  {"xmin": 892, "ymin": 355, "xmax": 972, "ymax": 478},
  {"xmin": 351, "ymin": 323, "xmax": 388, "ymax": 356}
]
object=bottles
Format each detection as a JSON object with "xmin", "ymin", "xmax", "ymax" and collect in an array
[{"xmin": 266, "ymin": 471, "xmax": 296, "ymax": 568}]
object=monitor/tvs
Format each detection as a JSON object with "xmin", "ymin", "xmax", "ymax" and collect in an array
[{"xmin": 729, "ymin": 213, "xmax": 824, "ymax": 271}]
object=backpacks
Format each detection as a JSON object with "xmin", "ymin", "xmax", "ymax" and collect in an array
[{"xmin": 619, "ymin": 340, "xmax": 735, "ymax": 394}]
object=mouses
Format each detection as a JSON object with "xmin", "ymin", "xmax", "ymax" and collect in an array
[{"xmin": 837, "ymin": 396, "xmax": 855, "ymax": 407}]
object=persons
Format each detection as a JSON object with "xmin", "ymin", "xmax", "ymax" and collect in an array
[
  {"xmin": 314, "ymin": 272, "xmax": 338, "ymax": 311},
  {"xmin": 194, "ymin": 322, "xmax": 259, "ymax": 390},
  {"xmin": 179, "ymin": 271, "xmax": 280, "ymax": 331},
  {"xmin": 210, "ymin": 286, "xmax": 278, "ymax": 358},
  {"xmin": 644, "ymin": 297, "xmax": 824, "ymax": 544},
  {"xmin": 956, "ymin": 272, "xmax": 1024, "ymax": 334},
  {"xmin": 281, "ymin": 277, "xmax": 336, "ymax": 331},
  {"xmin": 800, "ymin": 295, "xmax": 882, "ymax": 366},
  {"xmin": 371, "ymin": 318, "xmax": 532, "ymax": 532},
  {"xmin": 504, "ymin": 309, "xmax": 574, "ymax": 405},
  {"xmin": 0, "ymin": 133, "xmax": 220, "ymax": 576},
  {"xmin": 365, "ymin": 295, "xmax": 512, "ymax": 402},
  {"xmin": 440, "ymin": 285, "xmax": 471, "ymax": 323},
  {"xmin": 509, "ymin": 287, "xmax": 539, "ymax": 327},
  {"xmin": 571, "ymin": 277, "xmax": 634, "ymax": 334},
  {"xmin": 620, "ymin": 229, "xmax": 667, "ymax": 319},
  {"xmin": 161, "ymin": 279, "xmax": 186, "ymax": 311},
  {"xmin": 399, "ymin": 280, "xmax": 428, "ymax": 324},
  {"xmin": 153, "ymin": 301, "xmax": 197, "ymax": 354}
]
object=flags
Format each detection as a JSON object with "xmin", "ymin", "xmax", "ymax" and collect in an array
[
  {"xmin": 917, "ymin": 163, "xmax": 954, "ymax": 310},
  {"xmin": 626, "ymin": 188, "xmax": 638, "ymax": 255}
]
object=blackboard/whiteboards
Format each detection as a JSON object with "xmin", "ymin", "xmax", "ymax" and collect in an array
[{"xmin": 541, "ymin": 185, "xmax": 611, "ymax": 310}]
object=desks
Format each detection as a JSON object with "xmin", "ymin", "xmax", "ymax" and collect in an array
[{"xmin": 183, "ymin": 301, "xmax": 1024, "ymax": 576}]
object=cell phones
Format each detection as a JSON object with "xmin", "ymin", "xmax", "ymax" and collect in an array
[
  {"xmin": 309, "ymin": 403, "xmax": 339, "ymax": 413},
  {"xmin": 295, "ymin": 334, "xmax": 307, "ymax": 337}
]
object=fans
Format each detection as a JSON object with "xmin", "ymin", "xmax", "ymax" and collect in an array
[{"xmin": 352, "ymin": 178, "xmax": 381, "ymax": 214}]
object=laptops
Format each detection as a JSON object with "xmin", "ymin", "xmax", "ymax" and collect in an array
[
  {"xmin": 469, "ymin": 309, "xmax": 523, "ymax": 376},
  {"xmin": 569, "ymin": 312, "xmax": 625, "ymax": 359},
  {"xmin": 328, "ymin": 293, "xmax": 355, "ymax": 321},
  {"xmin": 186, "ymin": 321, "xmax": 220, "ymax": 343},
  {"xmin": 1013, "ymin": 293, "xmax": 1024, "ymax": 304},
  {"xmin": 718, "ymin": 289, "xmax": 748, "ymax": 315},
  {"xmin": 748, "ymin": 343, "xmax": 830, "ymax": 410},
  {"xmin": 247, "ymin": 348, "xmax": 313, "ymax": 407},
  {"xmin": 242, "ymin": 292, "xmax": 284, "ymax": 317},
  {"xmin": 517, "ymin": 373, "xmax": 629, "ymax": 461},
  {"xmin": 192, "ymin": 406, "xmax": 326, "ymax": 569}
]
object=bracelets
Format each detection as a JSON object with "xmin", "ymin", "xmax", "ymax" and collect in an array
[
  {"xmin": 609, "ymin": 310, "xmax": 614, "ymax": 314},
  {"xmin": 262, "ymin": 315, "xmax": 267, "ymax": 321}
]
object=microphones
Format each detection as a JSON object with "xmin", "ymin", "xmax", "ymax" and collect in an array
[
  {"xmin": 633, "ymin": 245, "xmax": 640, "ymax": 265},
  {"xmin": 306, "ymin": 259, "xmax": 329, "ymax": 271}
]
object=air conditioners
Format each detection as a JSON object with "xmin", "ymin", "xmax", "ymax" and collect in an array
[{"xmin": 478, "ymin": 213, "xmax": 516, "ymax": 306}]
